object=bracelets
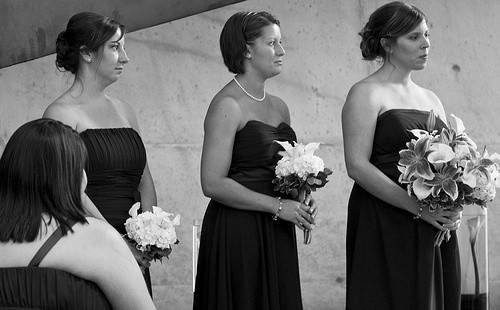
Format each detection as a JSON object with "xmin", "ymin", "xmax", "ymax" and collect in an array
[
  {"xmin": 272, "ymin": 197, "xmax": 282, "ymax": 220},
  {"xmin": 414, "ymin": 203, "xmax": 425, "ymax": 219}
]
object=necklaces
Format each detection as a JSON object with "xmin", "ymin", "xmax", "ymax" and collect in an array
[{"xmin": 233, "ymin": 75, "xmax": 266, "ymax": 103}]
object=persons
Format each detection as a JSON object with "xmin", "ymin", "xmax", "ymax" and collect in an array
[
  {"xmin": 338, "ymin": 0, "xmax": 464, "ymax": 310},
  {"xmin": 42, "ymin": 12, "xmax": 156, "ymax": 300},
  {"xmin": 0, "ymin": 117, "xmax": 158, "ymax": 310},
  {"xmin": 194, "ymin": 10, "xmax": 316, "ymax": 310}
]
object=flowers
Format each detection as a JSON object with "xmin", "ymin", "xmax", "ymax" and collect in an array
[
  {"xmin": 395, "ymin": 109, "xmax": 500, "ymax": 246},
  {"xmin": 274, "ymin": 140, "xmax": 332, "ymax": 244},
  {"xmin": 125, "ymin": 201, "xmax": 181, "ymax": 264}
]
